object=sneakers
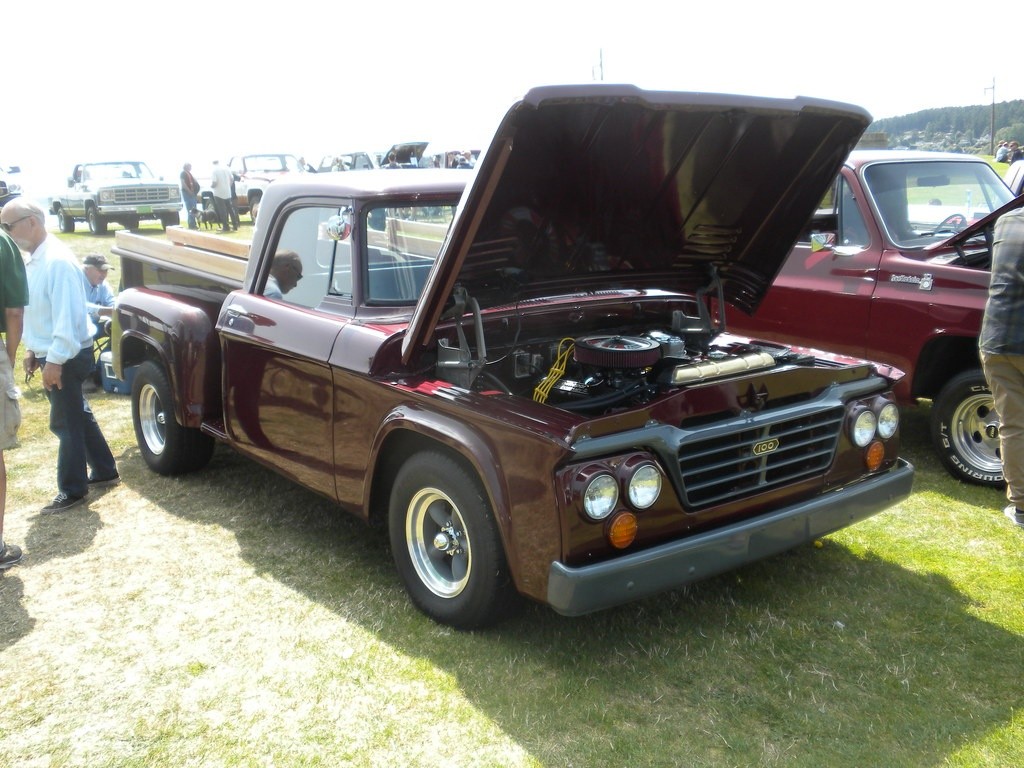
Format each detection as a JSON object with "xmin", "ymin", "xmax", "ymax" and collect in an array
[
  {"xmin": 192, "ymin": 226, "xmax": 201, "ymax": 231},
  {"xmin": 88, "ymin": 473, "xmax": 122, "ymax": 488},
  {"xmin": 40, "ymin": 488, "xmax": 91, "ymax": 515},
  {"xmin": 217, "ymin": 227, "xmax": 237, "ymax": 232},
  {"xmin": 0, "ymin": 536, "xmax": 23, "ymax": 570}
]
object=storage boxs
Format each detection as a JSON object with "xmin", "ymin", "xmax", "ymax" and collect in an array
[{"xmin": 101, "ymin": 351, "xmax": 140, "ymax": 394}]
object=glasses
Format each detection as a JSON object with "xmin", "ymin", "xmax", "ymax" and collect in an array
[
  {"xmin": 25, "ymin": 369, "xmax": 35, "ymax": 390},
  {"xmin": 0, "ymin": 216, "xmax": 33, "ymax": 230}
]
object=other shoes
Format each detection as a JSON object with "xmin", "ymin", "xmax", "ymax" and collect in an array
[{"xmin": 1001, "ymin": 501, "xmax": 1024, "ymax": 522}]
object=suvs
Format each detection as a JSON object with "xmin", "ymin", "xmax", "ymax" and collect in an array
[{"xmin": 702, "ymin": 151, "xmax": 1013, "ymax": 490}]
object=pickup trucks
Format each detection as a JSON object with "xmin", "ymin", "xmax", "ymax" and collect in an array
[
  {"xmin": 107, "ymin": 82, "xmax": 917, "ymax": 636},
  {"xmin": 194, "ymin": 151, "xmax": 310, "ymax": 224}
]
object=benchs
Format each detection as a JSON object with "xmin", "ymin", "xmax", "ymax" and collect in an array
[{"xmin": 295, "ymin": 263, "xmax": 433, "ymax": 307}]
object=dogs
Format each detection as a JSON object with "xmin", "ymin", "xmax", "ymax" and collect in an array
[{"xmin": 189, "ymin": 207, "xmax": 221, "ymax": 229}]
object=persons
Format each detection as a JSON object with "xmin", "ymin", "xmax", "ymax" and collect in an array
[
  {"xmin": 80, "ymin": 252, "xmax": 116, "ymax": 391},
  {"xmin": 0, "ymin": 228, "xmax": 29, "ymax": 569},
  {"xmin": 263, "ymin": 250, "xmax": 303, "ymax": 302},
  {"xmin": 180, "ymin": 145, "xmax": 477, "ymax": 234},
  {"xmin": 0, "ymin": 196, "xmax": 121, "ymax": 514},
  {"xmin": 995, "ymin": 140, "xmax": 1024, "ymax": 165},
  {"xmin": 977, "ymin": 207, "xmax": 1024, "ymax": 528}
]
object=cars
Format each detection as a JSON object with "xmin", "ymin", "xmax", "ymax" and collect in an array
[
  {"xmin": 907, "ymin": 160, "xmax": 1024, "ymax": 228},
  {"xmin": 317, "ymin": 141, "xmax": 480, "ymax": 171},
  {"xmin": 48, "ymin": 159, "xmax": 184, "ymax": 235}
]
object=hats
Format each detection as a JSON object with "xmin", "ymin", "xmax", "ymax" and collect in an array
[{"xmin": 85, "ymin": 253, "xmax": 116, "ymax": 272}]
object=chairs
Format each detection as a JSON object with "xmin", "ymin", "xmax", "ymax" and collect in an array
[{"xmin": 91, "ymin": 326, "xmax": 111, "ymax": 352}]
